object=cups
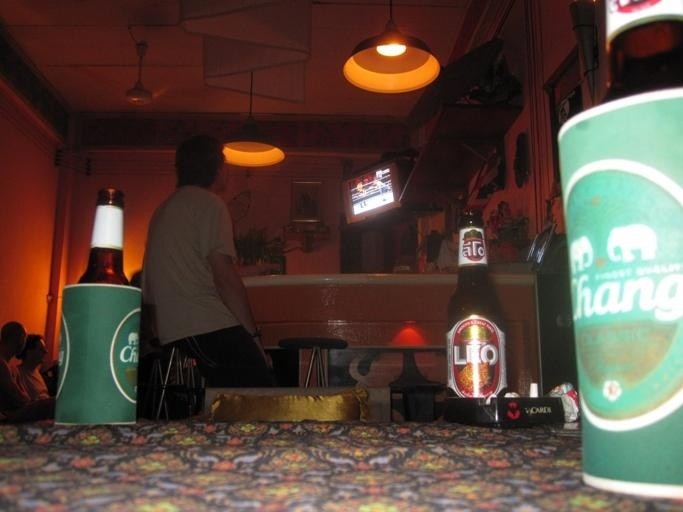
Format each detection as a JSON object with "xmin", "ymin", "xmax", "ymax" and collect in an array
[
  {"xmin": 54, "ymin": 284, "xmax": 143, "ymax": 425},
  {"xmin": 556, "ymin": 86, "xmax": 683, "ymax": 502}
]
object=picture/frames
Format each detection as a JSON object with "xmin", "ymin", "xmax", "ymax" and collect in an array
[{"xmin": 290, "ymin": 179, "xmax": 322, "ymax": 223}]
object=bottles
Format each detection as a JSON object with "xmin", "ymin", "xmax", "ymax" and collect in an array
[
  {"xmin": 447, "ymin": 208, "xmax": 510, "ymax": 398},
  {"xmin": 73, "ymin": 188, "xmax": 137, "ymax": 287},
  {"xmin": 597, "ymin": 0, "xmax": 682, "ymax": 103}
]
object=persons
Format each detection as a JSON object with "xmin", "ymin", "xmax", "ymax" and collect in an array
[
  {"xmin": 526, "ymin": 180, "xmax": 577, "ymax": 400},
  {"xmin": 437, "ymin": 223, "xmax": 464, "ymax": 275},
  {"xmin": 139, "ymin": 130, "xmax": 277, "ymax": 388},
  {"xmin": 372, "ymin": 176, "xmax": 389, "ymax": 202},
  {"xmin": 129, "ymin": 270, "xmax": 202, "ymax": 421},
  {"xmin": 356, "ymin": 179, "xmax": 368, "ymax": 208},
  {"xmin": 559, "ymin": 99, "xmax": 569, "ymax": 126},
  {"xmin": 0, "ymin": 322, "xmax": 56, "ymax": 425}
]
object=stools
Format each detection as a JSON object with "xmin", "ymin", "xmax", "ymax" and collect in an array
[
  {"xmin": 278, "ymin": 337, "xmax": 348, "ymax": 388},
  {"xmin": 141, "ymin": 337, "xmax": 206, "ymax": 420}
]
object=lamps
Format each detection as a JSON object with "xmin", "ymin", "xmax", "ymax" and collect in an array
[
  {"xmin": 222, "ymin": 69, "xmax": 286, "ymax": 168},
  {"xmin": 343, "ymin": 0, "xmax": 441, "ymax": 95},
  {"xmin": 124, "ymin": 39, "xmax": 152, "ymax": 106}
]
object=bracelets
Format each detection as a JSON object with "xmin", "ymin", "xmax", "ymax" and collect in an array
[{"xmin": 249, "ymin": 331, "xmax": 263, "ymax": 337}]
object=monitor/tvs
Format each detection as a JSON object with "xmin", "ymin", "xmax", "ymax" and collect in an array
[{"xmin": 342, "ymin": 162, "xmax": 402, "ymax": 225}]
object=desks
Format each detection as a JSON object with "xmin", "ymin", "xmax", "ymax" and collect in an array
[{"xmin": 343, "ymin": 345, "xmax": 449, "ymax": 421}]
